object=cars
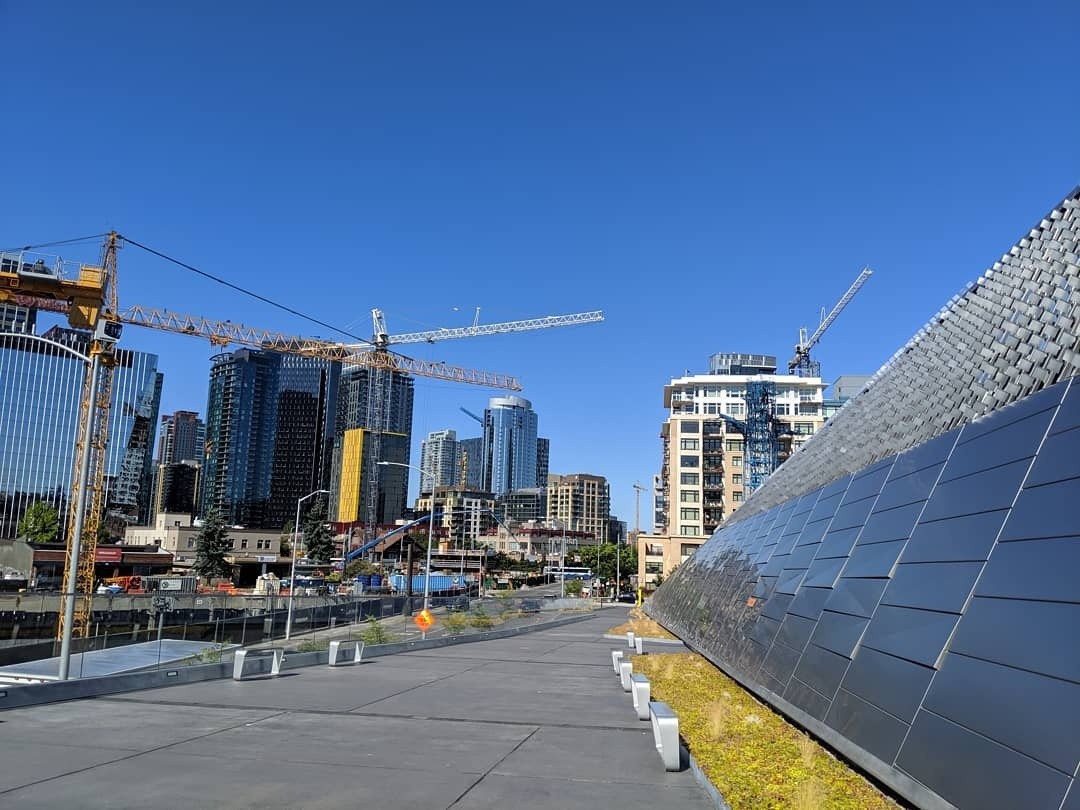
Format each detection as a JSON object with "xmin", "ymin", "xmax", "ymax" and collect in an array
[{"xmin": 614, "ymin": 592, "xmax": 644, "ymax": 603}]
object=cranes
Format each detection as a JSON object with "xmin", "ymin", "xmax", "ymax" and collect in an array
[
  {"xmin": 1, "ymin": 229, "xmax": 523, "ymax": 643},
  {"xmin": 633, "ymin": 478, "xmax": 649, "ymax": 534},
  {"xmin": 787, "ymin": 266, "xmax": 875, "ymax": 371},
  {"xmin": 299, "ymin": 306, "xmax": 605, "ymax": 353}
]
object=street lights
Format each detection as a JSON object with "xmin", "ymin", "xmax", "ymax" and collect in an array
[
  {"xmin": 286, "ymin": 489, "xmax": 331, "ymax": 640},
  {"xmin": 377, "ymin": 460, "xmax": 437, "ymax": 640}
]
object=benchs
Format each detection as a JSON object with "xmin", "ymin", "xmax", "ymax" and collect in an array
[
  {"xmin": 233, "ymin": 647, "xmax": 285, "ymax": 682},
  {"xmin": 610, "ymin": 647, "xmax": 650, "ymax": 720},
  {"xmin": 329, "ymin": 639, "xmax": 365, "ymax": 666},
  {"xmin": 647, "ymin": 702, "xmax": 680, "ymax": 772},
  {"xmin": 627, "ymin": 630, "xmax": 643, "ymax": 655}
]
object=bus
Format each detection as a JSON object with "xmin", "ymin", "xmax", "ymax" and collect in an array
[
  {"xmin": 292, "ymin": 565, "xmax": 338, "ymax": 582},
  {"xmin": 543, "ymin": 566, "xmax": 593, "ymax": 580}
]
